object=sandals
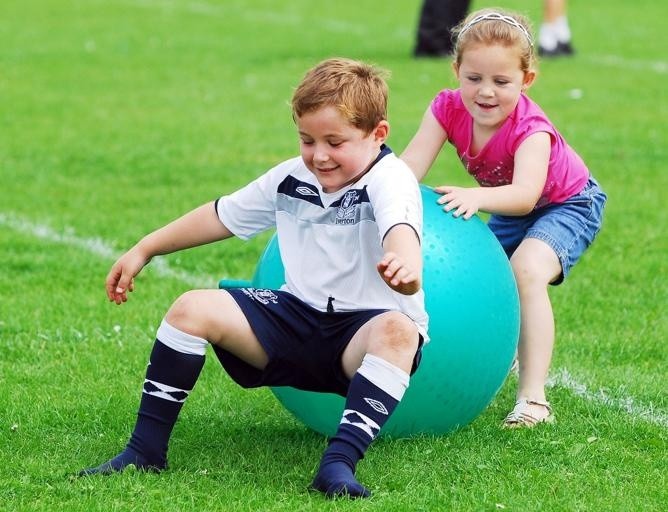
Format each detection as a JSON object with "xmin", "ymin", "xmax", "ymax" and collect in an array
[{"xmin": 498, "ymin": 398, "xmax": 556, "ymax": 430}]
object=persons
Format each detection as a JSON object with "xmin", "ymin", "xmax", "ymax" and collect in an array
[
  {"xmin": 77, "ymin": 56, "xmax": 430, "ymax": 502},
  {"xmin": 400, "ymin": 6, "xmax": 607, "ymax": 431},
  {"xmin": 414, "ymin": 0, "xmax": 471, "ymax": 59},
  {"xmin": 537, "ymin": 0, "xmax": 574, "ymax": 58}
]
